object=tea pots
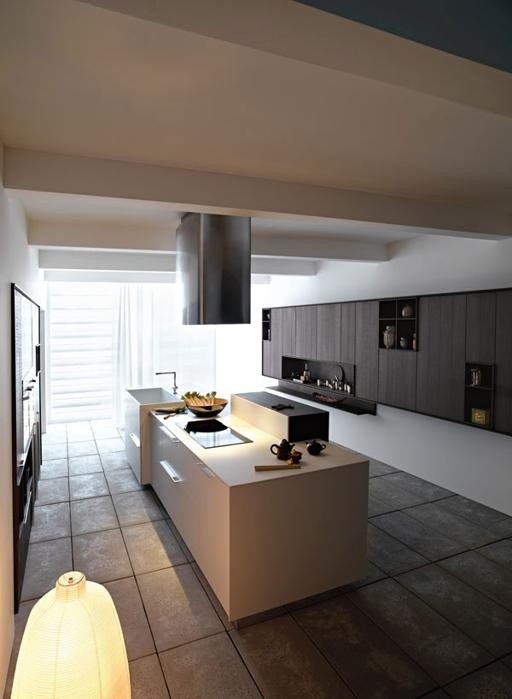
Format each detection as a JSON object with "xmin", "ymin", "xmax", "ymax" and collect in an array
[
  {"xmin": 270, "ymin": 439, "xmax": 295, "ymax": 460},
  {"xmin": 305, "ymin": 439, "xmax": 327, "ymax": 455}
]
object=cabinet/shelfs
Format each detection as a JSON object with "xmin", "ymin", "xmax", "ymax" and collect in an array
[
  {"xmin": 316, "ymin": 301, "xmax": 355, "ymax": 398},
  {"xmin": 262, "ymin": 308, "xmax": 271, "ymax": 377},
  {"xmin": 355, "ymin": 299, "xmax": 378, "ymax": 402},
  {"xmin": 464, "ymin": 289, "xmax": 495, "ymax": 432},
  {"xmin": 378, "ymin": 296, "xmax": 417, "ymax": 414},
  {"xmin": 271, "ymin": 308, "xmax": 282, "ymax": 379},
  {"xmin": 495, "ymin": 287, "xmax": 512, "ymax": 436},
  {"xmin": 416, "ymin": 291, "xmax": 466, "ymax": 424},
  {"xmin": 282, "ymin": 304, "xmax": 316, "ymax": 362}
]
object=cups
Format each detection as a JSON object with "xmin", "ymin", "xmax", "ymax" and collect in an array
[{"xmin": 290, "ymin": 451, "xmax": 302, "ymax": 461}]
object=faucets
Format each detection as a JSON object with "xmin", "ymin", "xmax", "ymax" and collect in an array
[{"xmin": 154, "ymin": 371, "xmax": 178, "ymax": 395}]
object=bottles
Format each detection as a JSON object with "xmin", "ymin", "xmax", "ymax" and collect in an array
[
  {"xmin": 316, "ymin": 378, "xmax": 350, "ymax": 392},
  {"xmin": 304, "ymin": 363, "xmax": 310, "ymax": 380},
  {"xmin": 305, "ymin": 375, "xmax": 309, "ymax": 383}
]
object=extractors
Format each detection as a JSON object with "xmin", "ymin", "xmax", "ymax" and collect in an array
[{"xmin": 175, "ymin": 215, "xmax": 251, "ymax": 325}]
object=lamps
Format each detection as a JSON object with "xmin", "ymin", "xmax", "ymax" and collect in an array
[{"xmin": 11, "ymin": 571, "xmax": 132, "ymax": 699}]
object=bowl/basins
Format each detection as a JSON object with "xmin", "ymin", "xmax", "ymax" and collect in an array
[{"xmin": 186, "ymin": 397, "xmax": 228, "ymax": 417}]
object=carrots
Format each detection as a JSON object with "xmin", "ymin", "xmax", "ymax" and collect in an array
[{"xmin": 185, "ymin": 395, "xmax": 215, "ymax": 406}]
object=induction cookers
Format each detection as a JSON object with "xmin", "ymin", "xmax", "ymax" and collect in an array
[{"xmin": 175, "ymin": 418, "xmax": 254, "ymax": 450}]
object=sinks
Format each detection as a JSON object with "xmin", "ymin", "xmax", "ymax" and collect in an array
[{"xmin": 126, "ymin": 387, "xmax": 180, "ymax": 405}]
